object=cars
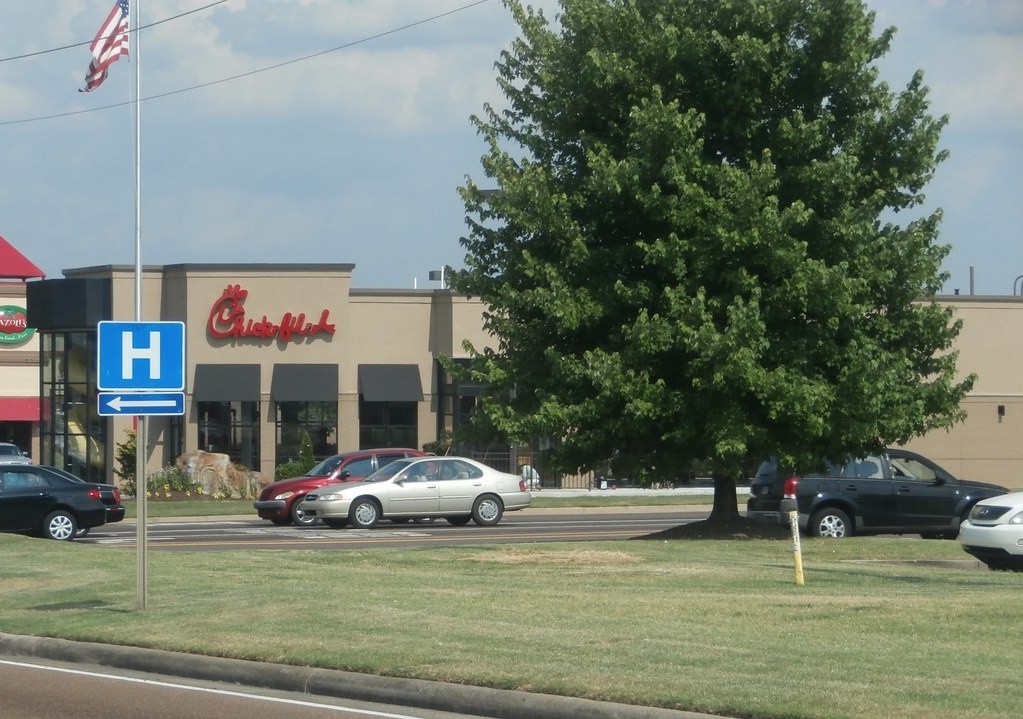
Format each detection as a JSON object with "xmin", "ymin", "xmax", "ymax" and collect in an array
[
  {"xmin": 254, "ymin": 447, "xmax": 437, "ymax": 526},
  {"xmin": 0, "ymin": 465, "xmax": 108, "ymax": 543},
  {"xmin": 958, "ymin": 491, "xmax": 1023, "ymax": 567},
  {"xmin": 298, "ymin": 455, "xmax": 532, "ymax": 529},
  {"xmin": 39, "ymin": 466, "xmax": 126, "ymax": 538}
]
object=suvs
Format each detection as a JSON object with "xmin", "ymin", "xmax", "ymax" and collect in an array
[
  {"xmin": 745, "ymin": 444, "xmax": 1012, "ymax": 538},
  {"xmin": 0, "ymin": 442, "xmax": 32, "ymax": 467}
]
